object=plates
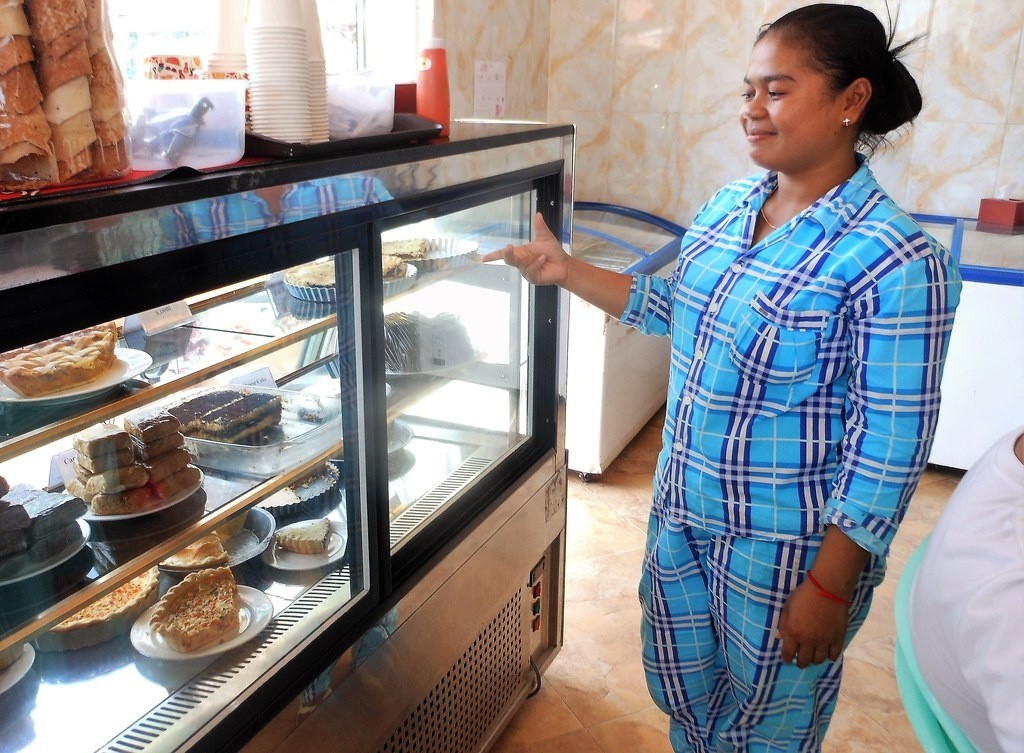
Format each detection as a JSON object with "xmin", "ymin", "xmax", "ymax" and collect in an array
[
  {"xmin": 0, "ymin": 518, "xmax": 92, "ymax": 586},
  {"xmin": 0, "ymin": 348, "xmax": 153, "ymax": 406},
  {"xmin": 35, "ymin": 566, "xmax": 161, "ymax": 652},
  {"xmin": 62, "ymin": 464, "xmax": 204, "ymax": 521},
  {"xmin": 256, "ymin": 461, "xmax": 340, "ymax": 517},
  {"xmin": 386, "ymin": 362, "xmax": 470, "ymax": 377},
  {"xmin": 0, "ymin": 642, "xmax": 36, "ymax": 694},
  {"xmin": 260, "ymin": 521, "xmax": 347, "ymax": 571},
  {"xmin": 299, "ymin": 378, "xmax": 391, "ymax": 411},
  {"xmin": 403, "ymin": 237, "xmax": 479, "ymax": 271},
  {"xmin": 382, "ymin": 263, "xmax": 418, "ymax": 296},
  {"xmin": 159, "ymin": 506, "xmax": 276, "ymax": 578},
  {"xmin": 329, "ymin": 421, "xmax": 414, "ymax": 461},
  {"xmin": 284, "ymin": 276, "xmax": 335, "ymax": 302},
  {"xmin": 130, "ymin": 584, "xmax": 275, "ymax": 661}
]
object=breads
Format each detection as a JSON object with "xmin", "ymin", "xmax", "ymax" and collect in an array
[
  {"xmin": 286, "ymin": 237, "xmax": 429, "ymax": 287},
  {"xmin": 0, "ymin": 322, "xmax": 118, "ymax": 398},
  {"xmin": 162, "ymin": 390, "xmax": 283, "ymax": 444},
  {"xmin": 31, "ymin": 509, "xmax": 330, "ymax": 651},
  {"xmin": 64, "ymin": 408, "xmax": 201, "ymax": 516},
  {"xmin": 0, "ymin": 476, "xmax": 87, "ymax": 578}
]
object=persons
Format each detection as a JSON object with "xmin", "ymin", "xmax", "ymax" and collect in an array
[
  {"xmin": 913, "ymin": 425, "xmax": 1024, "ymax": 752},
  {"xmin": 481, "ymin": 0, "xmax": 964, "ymax": 753}
]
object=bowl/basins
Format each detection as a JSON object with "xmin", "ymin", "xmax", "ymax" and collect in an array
[{"xmin": 123, "ymin": 79, "xmax": 248, "ymax": 169}]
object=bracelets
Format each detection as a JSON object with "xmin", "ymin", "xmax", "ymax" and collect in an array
[{"xmin": 807, "ymin": 570, "xmax": 850, "ymax": 606}]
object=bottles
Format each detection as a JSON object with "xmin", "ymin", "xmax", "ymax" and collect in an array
[{"xmin": 417, "ymin": 38, "xmax": 450, "ymax": 136}]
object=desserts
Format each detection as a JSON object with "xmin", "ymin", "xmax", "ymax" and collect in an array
[{"xmin": 383, "ymin": 311, "xmax": 419, "ymax": 371}]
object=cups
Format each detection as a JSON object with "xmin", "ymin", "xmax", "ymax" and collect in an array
[{"xmin": 144, "ymin": 0, "xmax": 329, "ymax": 143}]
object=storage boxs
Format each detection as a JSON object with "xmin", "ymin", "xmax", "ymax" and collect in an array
[{"xmin": 122, "ymin": 78, "xmax": 251, "ymax": 171}]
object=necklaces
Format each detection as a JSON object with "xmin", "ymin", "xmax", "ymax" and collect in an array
[{"xmin": 761, "ymin": 185, "xmax": 778, "ymax": 230}]
